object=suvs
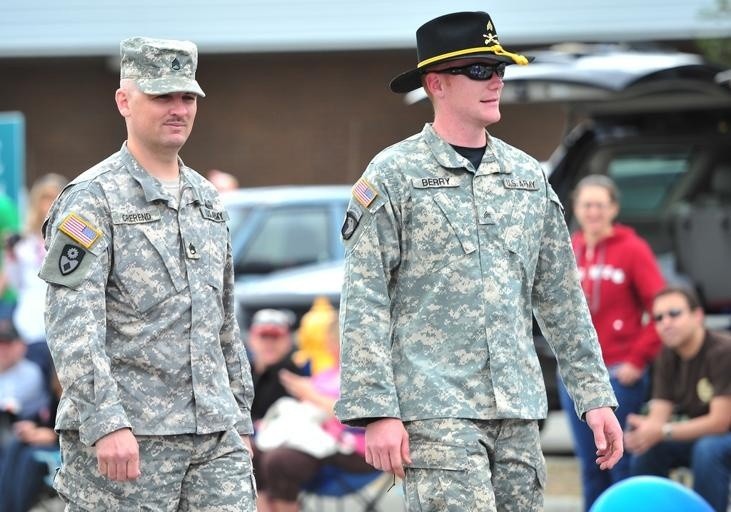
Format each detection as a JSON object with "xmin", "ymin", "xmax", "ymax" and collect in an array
[{"xmin": 405, "ymin": 41, "xmax": 730, "ymax": 455}]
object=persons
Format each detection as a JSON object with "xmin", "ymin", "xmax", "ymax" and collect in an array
[
  {"xmin": 558, "ymin": 168, "xmax": 675, "ymax": 509},
  {"xmin": 609, "ymin": 287, "xmax": 730, "ymax": 512},
  {"xmin": 2, "ymin": 315, "xmax": 49, "ymax": 424},
  {"xmin": 1, "ymin": 172, "xmax": 70, "ymax": 406},
  {"xmin": 240, "ymin": 312, "xmax": 410, "ymax": 512},
  {"xmin": 241, "ymin": 307, "xmax": 309, "ymax": 430},
  {"xmin": 0, "ymin": 356, "xmax": 65, "ymax": 511},
  {"xmin": 328, "ymin": 5, "xmax": 631, "ymax": 511},
  {"xmin": 35, "ymin": 30, "xmax": 268, "ymax": 512}
]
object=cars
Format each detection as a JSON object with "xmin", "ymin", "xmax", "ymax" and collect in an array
[{"xmin": 213, "ymin": 184, "xmax": 356, "ymax": 310}]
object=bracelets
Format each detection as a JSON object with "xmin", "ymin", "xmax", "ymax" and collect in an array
[{"xmin": 662, "ymin": 423, "xmax": 674, "ymax": 443}]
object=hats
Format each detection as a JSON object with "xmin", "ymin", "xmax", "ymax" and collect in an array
[
  {"xmin": 251, "ymin": 308, "xmax": 292, "ymax": 325},
  {"xmin": 389, "ymin": 12, "xmax": 536, "ymax": 94},
  {"xmin": 118, "ymin": 34, "xmax": 207, "ymax": 98}
]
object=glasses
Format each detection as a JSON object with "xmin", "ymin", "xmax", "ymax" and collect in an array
[
  {"xmin": 654, "ymin": 307, "xmax": 685, "ymax": 323},
  {"xmin": 425, "ymin": 61, "xmax": 506, "ymax": 80},
  {"xmin": 259, "ymin": 329, "xmax": 283, "ymax": 339}
]
